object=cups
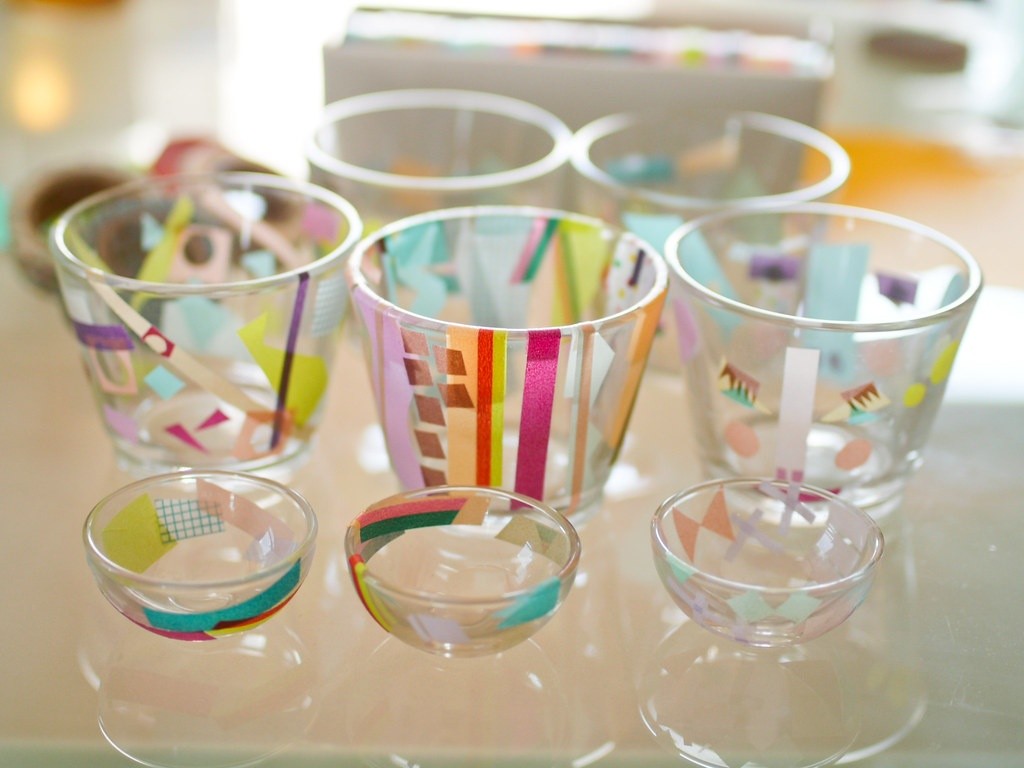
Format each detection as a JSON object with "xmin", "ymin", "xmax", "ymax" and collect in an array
[
  {"xmin": 84, "ymin": 469, "xmax": 317, "ymax": 641},
  {"xmin": 49, "ymin": 170, "xmax": 360, "ymax": 487},
  {"xmin": 652, "ymin": 478, "xmax": 885, "ymax": 646},
  {"xmin": 348, "ymin": 206, "xmax": 667, "ymax": 536},
  {"xmin": 570, "ymin": 106, "xmax": 849, "ymax": 371},
  {"xmin": 299, "ymin": 90, "xmax": 571, "ymax": 361},
  {"xmin": 665, "ymin": 202, "xmax": 982, "ymax": 530},
  {"xmin": 344, "ymin": 486, "xmax": 581, "ymax": 658}
]
